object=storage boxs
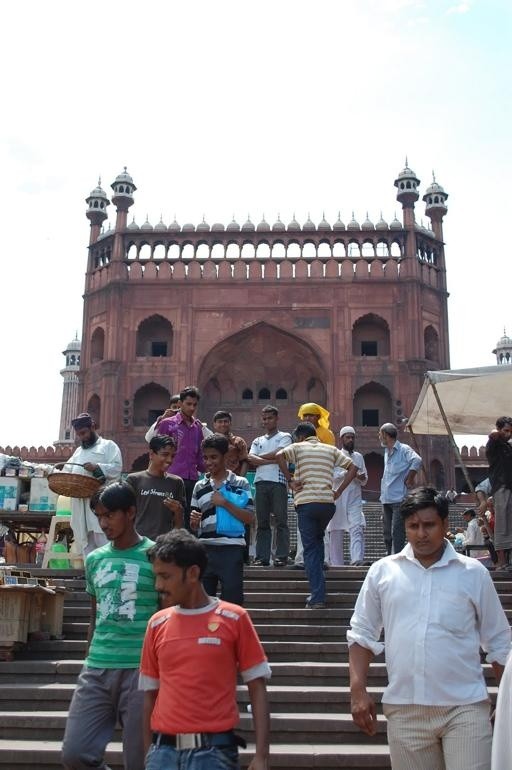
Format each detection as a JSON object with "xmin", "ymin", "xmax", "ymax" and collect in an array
[
  {"xmin": 29, "ymin": 477, "xmax": 58, "ymax": 512},
  {"xmin": 0, "ymin": 477, "xmax": 25, "ymax": 510}
]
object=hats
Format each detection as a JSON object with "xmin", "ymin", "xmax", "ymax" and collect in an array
[
  {"xmin": 462, "ymin": 508, "xmax": 476, "ymax": 515},
  {"xmin": 70, "ymin": 413, "xmax": 91, "ymax": 427},
  {"xmin": 339, "ymin": 426, "xmax": 355, "ymax": 438}
]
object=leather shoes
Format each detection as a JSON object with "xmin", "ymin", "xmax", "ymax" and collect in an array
[{"xmin": 252, "ymin": 559, "xmax": 286, "ymax": 566}]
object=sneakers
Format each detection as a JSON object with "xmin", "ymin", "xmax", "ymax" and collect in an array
[
  {"xmin": 352, "ymin": 561, "xmax": 369, "ymax": 566},
  {"xmin": 305, "ymin": 596, "xmax": 324, "ymax": 609}
]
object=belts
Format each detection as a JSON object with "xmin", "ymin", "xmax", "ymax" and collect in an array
[{"xmin": 151, "ymin": 731, "xmax": 246, "ymax": 752}]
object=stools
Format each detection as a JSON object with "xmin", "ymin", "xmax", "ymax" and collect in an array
[
  {"xmin": 42, "ymin": 516, "xmax": 83, "ymax": 569},
  {"xmin": 464, "ymin": 539, "xmax": 498, "ymax": 564}
]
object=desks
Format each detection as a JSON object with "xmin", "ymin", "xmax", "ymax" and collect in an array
[{"xmin": 0, "ymin": 509, "xmax": 56, "ymax": 545}]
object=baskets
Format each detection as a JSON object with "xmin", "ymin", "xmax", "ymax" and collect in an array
[{"xmin": 46, "ymin": 461, "xmax": 100, "ymax": 498}]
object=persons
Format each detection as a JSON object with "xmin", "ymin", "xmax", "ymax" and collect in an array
[
  {"xmin": 247, "ymin": 405, "xmax": 293, "ymax": 566},
  {"xmin": 345, "ymin": 487, "xmax": 512, "ymax": 770},
  {"xmin": 56, "ymin": 412, "xmax": 122, "ymax": 569},
  {"xmin": 138, "ymin": 527, "xmax": 272, "ymax": 770},
  {"xmin": 128, "ymin": 385, "xmax": 254, "ymax": 608},
  {"xmin": 446, "ymin": 416, "xmax": 511, "ymax": 571},
  {"xmin": 379, "ymin": 423, "xmax": 423, "ymax": 555},
  {"xmin": 287, "ymin": 403, "xmax": 336, "ymax": 570},
  {"xmin": 275, "ymin": 421, "xmax": 359, "ymax": 609},
  {"xmin": 327, "ymin": 425, "xmax": 368, "ymax": 565},
  {"xmin": 60, "ymin": 480, "xmax": 172, "ymax": 770}
]
